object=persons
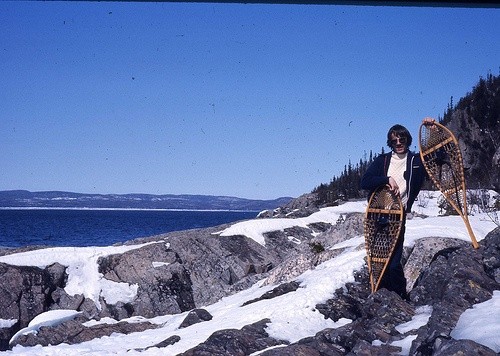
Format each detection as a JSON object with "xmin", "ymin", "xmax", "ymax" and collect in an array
[{"xmin": 360, "ymin": 117, "xmax": 443, "ymax": 303}]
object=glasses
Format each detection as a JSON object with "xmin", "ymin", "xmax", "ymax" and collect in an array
[{"xmin": 391, "ymin": 137, "xmax": 407, "ymax": 145}]
